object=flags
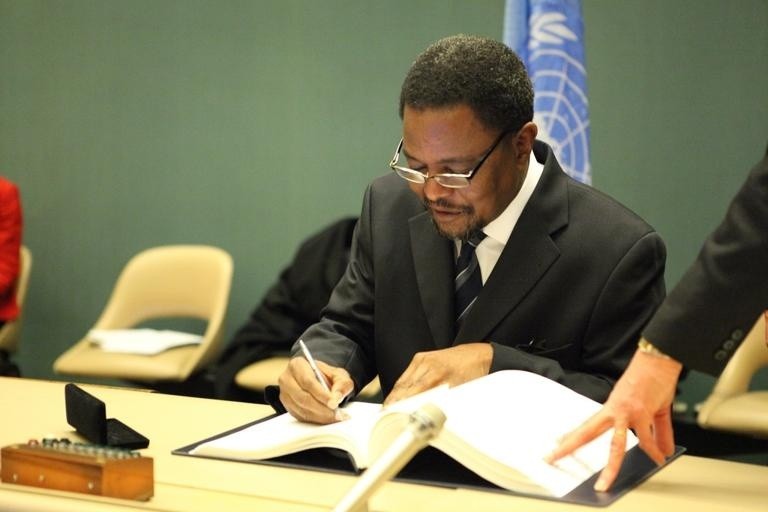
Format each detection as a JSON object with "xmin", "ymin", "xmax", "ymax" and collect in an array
[{"xmin": 501, "ymin": 0, "xmax": 592, "ymax": 188}]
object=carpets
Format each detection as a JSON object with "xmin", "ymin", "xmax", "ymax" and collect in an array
[{"xmin": 453, "ymin": 230, "xmax": 489, "ymax": 330}]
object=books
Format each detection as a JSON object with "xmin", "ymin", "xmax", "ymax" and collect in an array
[{"xmin": 171, "ymin": 361, "xmax": 686, "ymax": 507}]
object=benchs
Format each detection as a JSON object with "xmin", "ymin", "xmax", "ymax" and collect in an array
[{"xmin": 501, "ymin": 0, "xmax": 592, "ymax": 188}]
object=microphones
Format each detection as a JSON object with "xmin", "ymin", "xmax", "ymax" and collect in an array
[{"xmin": 236, "ymin": 342, "xmax": 385, "ymax": 400}]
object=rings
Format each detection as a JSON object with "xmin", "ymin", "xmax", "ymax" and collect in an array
[{"xmin": 299, "ymin": 340, "xmax": 340, "ymax": 420}]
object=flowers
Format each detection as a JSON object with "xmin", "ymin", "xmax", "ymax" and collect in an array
[{"xmin": 335, "ymin": 402, "xmax": 447, "ymax": 512}]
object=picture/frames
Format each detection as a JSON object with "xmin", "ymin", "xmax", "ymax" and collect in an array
[{"xmin": 0, "ymin": 373, "xmax": 768, "ymax": 512}]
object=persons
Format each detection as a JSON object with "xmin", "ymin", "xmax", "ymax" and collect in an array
[
  {"xmin": 545, "ymin": 151, "xmax": 767, "ymax": 493},
  {"xmin": 279, "ymin": 35, "xmax": 667, "ymax": 424},
  {"xmin": 0, "ymin": 177, "xmax": 22, "ymax": 319}
]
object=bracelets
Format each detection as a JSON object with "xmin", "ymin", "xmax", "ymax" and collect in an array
[{"xmin": 637, "ymin": 337, "xmax": 670, "ymax": 359}]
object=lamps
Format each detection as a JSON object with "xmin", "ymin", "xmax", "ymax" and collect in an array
[
  {"xmin": 53, "ymin": 243, "xmax": 236, "ymax": 386},
  {"xmin": 693, "ymin": 310, "xmax": 767, "ymax": 435}
]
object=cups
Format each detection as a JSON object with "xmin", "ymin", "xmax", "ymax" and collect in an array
[{"xmin": 389, "ymin": 136, "xmax": 502, "ymax": 188}]
